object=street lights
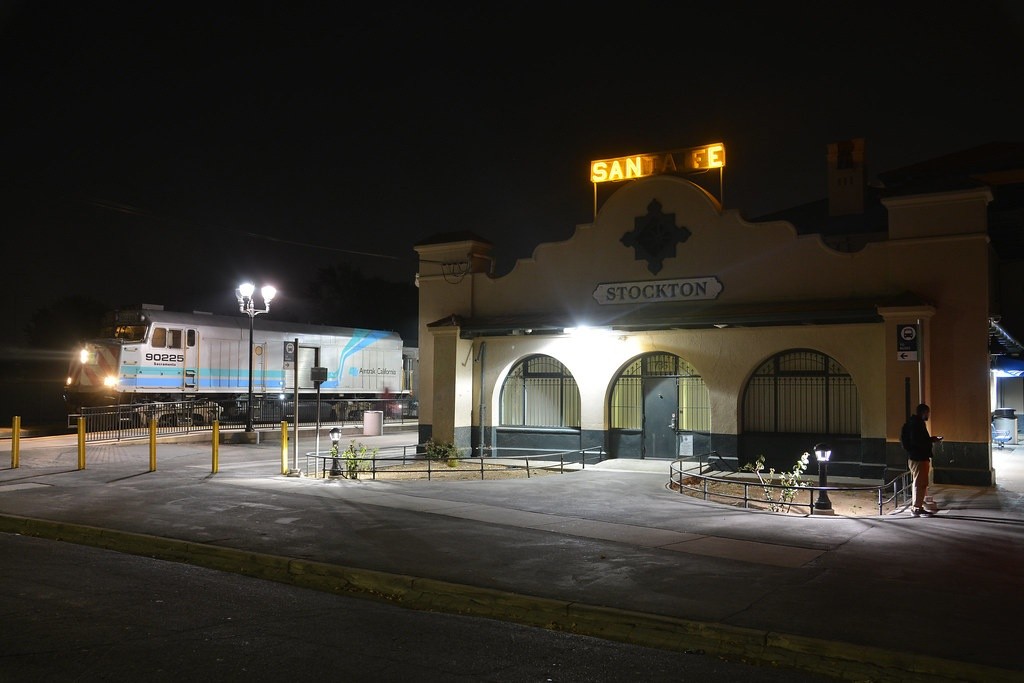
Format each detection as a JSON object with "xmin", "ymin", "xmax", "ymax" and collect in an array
[{"xmin": 235, "ymin": 282, "xmax": 277, "ymax": 431}]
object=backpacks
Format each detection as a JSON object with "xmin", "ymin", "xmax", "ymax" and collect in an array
[{"xmin": 901, "ymin": 421, "xmax": 924, "ymax": 450}]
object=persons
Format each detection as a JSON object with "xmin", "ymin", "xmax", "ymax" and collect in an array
[{"xmin": 907, "ymin": 404, "xmax": 944, "ymax": 518}]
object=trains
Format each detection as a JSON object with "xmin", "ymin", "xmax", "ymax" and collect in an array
[{"xmin": 63, "ymin": 303, "xmax": 418, "ymax": 422}]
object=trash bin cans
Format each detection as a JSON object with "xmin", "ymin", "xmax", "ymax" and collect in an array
[
  {"xmin": 363, "ymin": 411, "xmax": 383, "ymax": 436},
  {"xmin": 993, "ymin": 408, "xmax": 1018, "ymax": 445}
]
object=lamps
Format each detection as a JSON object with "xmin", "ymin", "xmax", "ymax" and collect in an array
[
  {"xmin": 814, "ymin": 443, "xmax": 833, "ymax": 509},
  {"xmin": 329, "ymin": 427, "xmax": 343, "ymax": 476}
]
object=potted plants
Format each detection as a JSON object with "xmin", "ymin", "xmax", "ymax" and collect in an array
[{"xmin": 423, "ymin": 435, "xmax": 465, "ymax": 468}]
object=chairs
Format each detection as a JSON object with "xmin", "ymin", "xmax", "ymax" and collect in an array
[{"xmin": 991, "ymin": 421, "xmax": 1012, "ymax": 450}]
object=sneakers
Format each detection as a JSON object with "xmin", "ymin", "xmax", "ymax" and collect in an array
[{"xmin": 911, "ymin": 506, "xmax": 933, "ymax": 518}]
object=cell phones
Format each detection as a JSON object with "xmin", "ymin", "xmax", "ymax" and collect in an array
[{"xmin": 937, "ymin": 437, "xmax": 943, "ymax": 440}]
